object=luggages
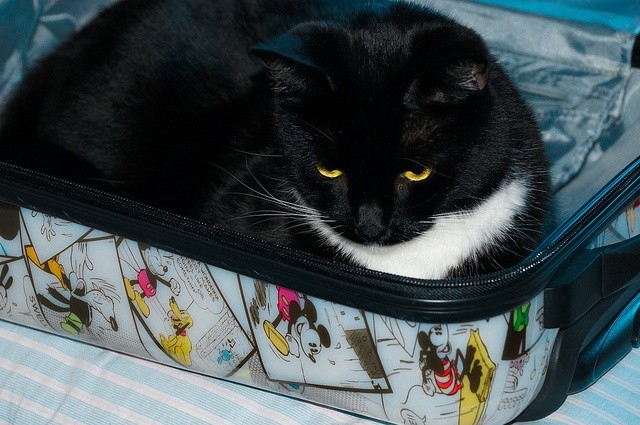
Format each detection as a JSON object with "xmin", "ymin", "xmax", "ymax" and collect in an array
[{"xmin": 0, "ymin": 0, "xmax": 639, "ymax": 425}]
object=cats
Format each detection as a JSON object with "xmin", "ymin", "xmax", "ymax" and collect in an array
[{"xmin": 1, "ymin": 1, "xmax": 553, "ymax": 286}]
